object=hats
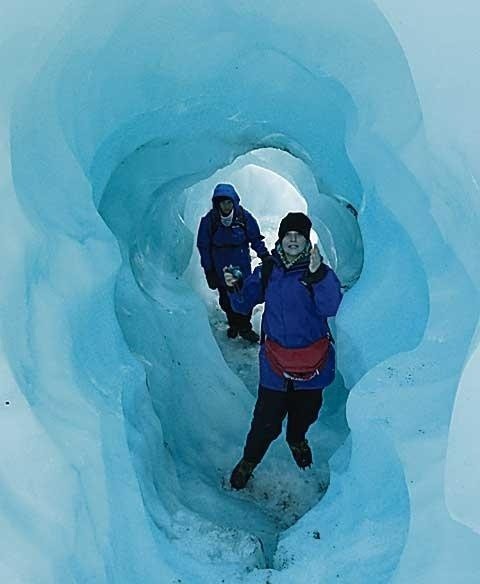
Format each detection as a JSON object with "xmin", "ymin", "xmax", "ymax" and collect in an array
[{"xmin": 278, "ymin": 213, "xmax": 312, "ymax": 243}]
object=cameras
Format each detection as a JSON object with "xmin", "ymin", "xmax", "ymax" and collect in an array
[{"xmin": 225, "ymin": 265, "xmax": 243, "ymax": 280}]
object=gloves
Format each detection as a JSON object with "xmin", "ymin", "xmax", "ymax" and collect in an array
[{"xmin": 204, "ymin": 269, "xmax": 218, "ymax": 289}]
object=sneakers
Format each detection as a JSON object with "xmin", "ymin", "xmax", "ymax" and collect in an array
[
  {"xmin": 287, "ymin": 439, "xmax": 312, "ymax": 468},
  {"xmin": 230, "ymin": 457, "xmax": 257, "ymax": 489}
]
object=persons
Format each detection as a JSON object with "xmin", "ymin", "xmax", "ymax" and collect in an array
[
  {"xmin": 222, "ymin": 212, "xmax": 343, "ymax": 489},
  {"xmin": 197, "ymin": 184, "xmax": 270, "ymax": 343}
]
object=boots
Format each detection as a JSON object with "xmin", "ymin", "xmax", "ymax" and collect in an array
[
  {"xmin": 227, "ymin": 320, "xmax": 239, "ymax": 339},
  {"xmin": 238, "ymin": 325, "xmax": 259, "ymax": 342}
]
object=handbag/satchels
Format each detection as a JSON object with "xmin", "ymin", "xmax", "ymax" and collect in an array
[{"xmin": 263, "ymin": 337, "xmax": 329, "ymax": 381}]
